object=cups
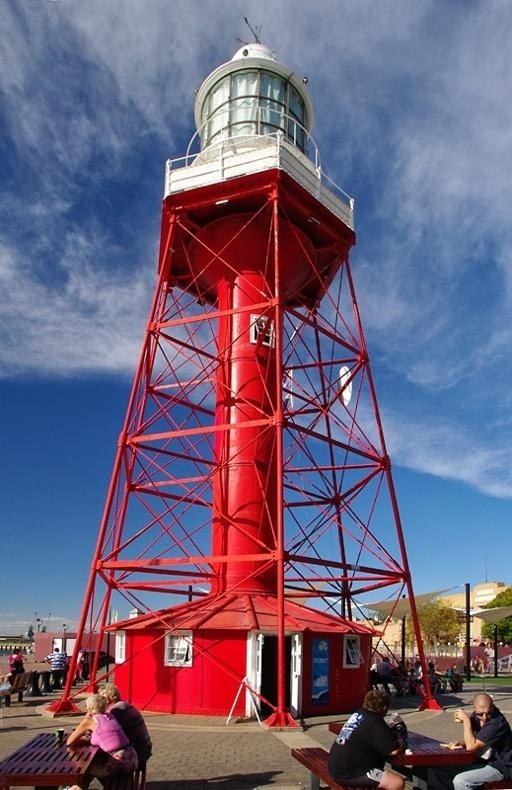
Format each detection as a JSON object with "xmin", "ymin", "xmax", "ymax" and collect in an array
[{"xmin": 55, "ymin": 729, "xmax": 65, "ymax": 739}]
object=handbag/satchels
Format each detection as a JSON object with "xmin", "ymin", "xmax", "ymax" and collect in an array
[
  {"xmin": 10, "ymin": 660, "xmax": 23, "ymax": 668},
  {"xmin": 386, "ymin": 713, "xmax": 407, "ymax": 740}
]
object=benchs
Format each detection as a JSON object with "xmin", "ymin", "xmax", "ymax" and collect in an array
[
  {"xmin": 101, "ymin": 757, "xmax": 147, "ymax": 790},
  {"xmin": 290, "ymin": 747, "xmax": 380, "ymax": 790},
  {"xmin": 0, "ymin": 670, "xmax": 34, "ymax": 707}
]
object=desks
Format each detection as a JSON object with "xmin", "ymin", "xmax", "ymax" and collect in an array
[
  {"xmin": 0, "ymin": 731, "xmax": 101, "ymax": 790},
  {"xmin": 328, "ymin": 721, "xmax": 477, "ymax": 790}
]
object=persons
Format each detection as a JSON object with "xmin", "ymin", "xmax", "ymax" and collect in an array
[
  {"xmin": 99, "ymin": 683, "xmax": 153, "ymax": 761},
  {"xmin": 448, "ymin": 693, "xmax": 512, "ymax": 790},
  {"xmin": 328, "ymin": 690, "xmax": 407, "ymax": 790},
  {"xmin": 67, "ymin": 694, "xmax": 138, "ymax": 790},
  {"xmin": 8, "ymin": 648, "xmax": 85, "ymax": 689},
  {"xmin": 372, "ymin": 641, "xmax": 495, "ymax": 695}
]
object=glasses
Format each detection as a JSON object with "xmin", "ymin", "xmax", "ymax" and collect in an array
[{"xmin": 476, "ymin": 711, "xmax": 494, "ymax": 716}]
